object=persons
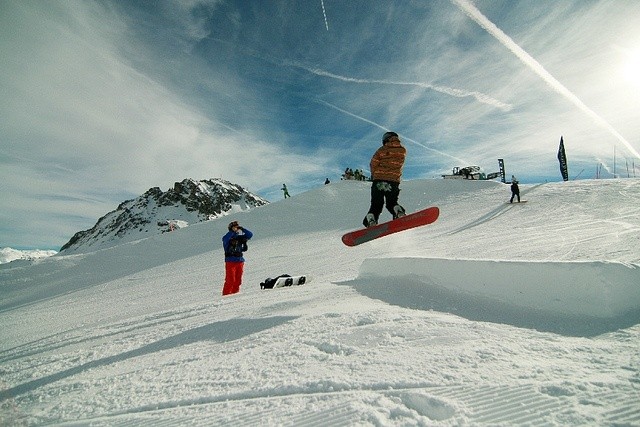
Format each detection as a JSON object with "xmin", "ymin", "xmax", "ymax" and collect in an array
[
  {"xmin": 363, "ymin": 132, "xmax": 407, "ymax": 228},
  {"xmin": 222, "ymin": 222, "xmax": 253, "ymax": 295},
  {"xmin": 510, "ymin": 179, "xmax": 521, "ymax": 203},
  {"xmin": 281, "ymin": 184, "xmax": 291, "ymax": 199},
  {"xmin": 345, "ymin": 168, "xmax": 365, "ymax": 181}
]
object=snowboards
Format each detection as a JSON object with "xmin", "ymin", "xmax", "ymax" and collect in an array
[
  {"xmin": 272, "ymin": 274, "xmax": 307, "ymax": 288},
  {"xmin": 506, "ymin": 200, "xmax": 527, "ymax": 203},
  {"xmin": 341, "ymin": 207, "xmax": 440, "ymax": 247}
]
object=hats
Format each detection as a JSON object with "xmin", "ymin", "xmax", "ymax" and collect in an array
[
  {"xmin": 382, "ymin": 131, "xmax": 398, "ymax": 143},
  {"xmin": 228, "ymin": 220, "xmax": 238, "ymax": 231}
]
